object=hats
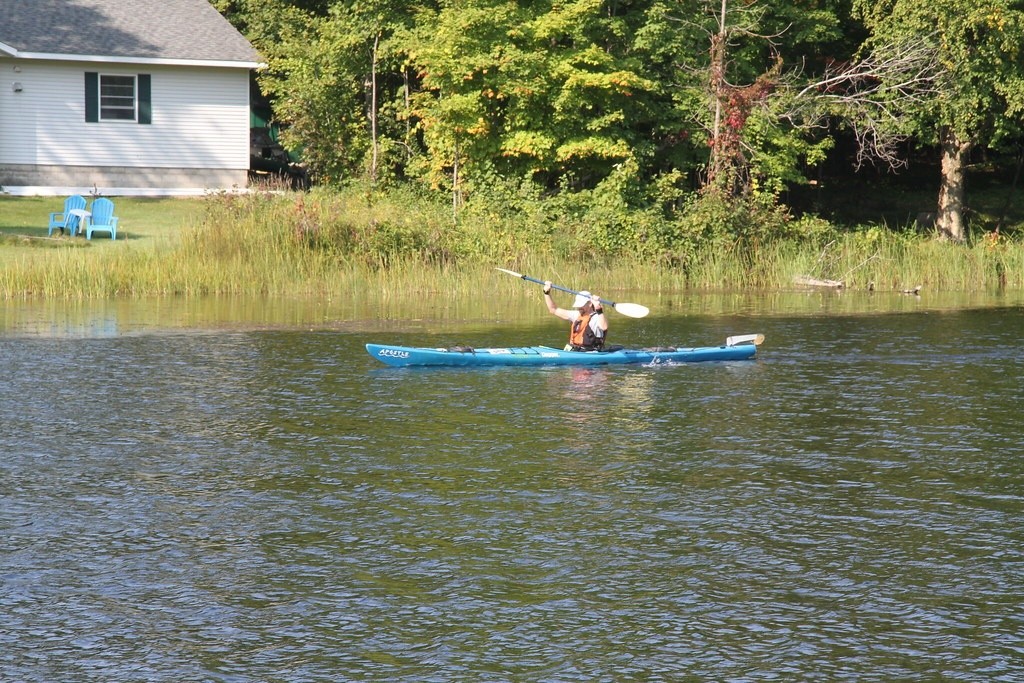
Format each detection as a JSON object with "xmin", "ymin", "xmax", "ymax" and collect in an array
[{"xmin": 572, "ymin": 291, "xmax": 592, "ymax": 307}]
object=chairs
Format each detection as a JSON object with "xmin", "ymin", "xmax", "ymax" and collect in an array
[
  {"xmin": 48, "ymin": 193, "xmax": 86, "ymax": 237},
  {"xmin": 85, "ymin": 198, "xmax": 118, "ymax": 239}
]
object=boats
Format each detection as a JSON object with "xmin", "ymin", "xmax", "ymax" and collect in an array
[{"xmin": 365, "ymin": 334, "xmax": 765, "ymax": 366}]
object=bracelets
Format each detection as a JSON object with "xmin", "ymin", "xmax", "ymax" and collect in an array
[
  {"xmin": 543, "ymin": 290, "xmax": 550, "ymax": 295},
  {"xmin": 596, "ymin": 308, "xmax": 603, "ymax": 316}
]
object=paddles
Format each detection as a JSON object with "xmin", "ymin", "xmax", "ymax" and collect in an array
[{"xmin": 493, "ymin": 266, "xmax": 650, "ymax": 320}]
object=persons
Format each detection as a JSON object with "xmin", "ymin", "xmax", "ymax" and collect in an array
[{"xmin": 542, "ymin": 280, "xmax": 608, "ymax": 353}]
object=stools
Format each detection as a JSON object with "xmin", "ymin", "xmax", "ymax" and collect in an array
[{"xmin": 63, "ymin": 208, "xmax": 92, "ymax": 233}]
